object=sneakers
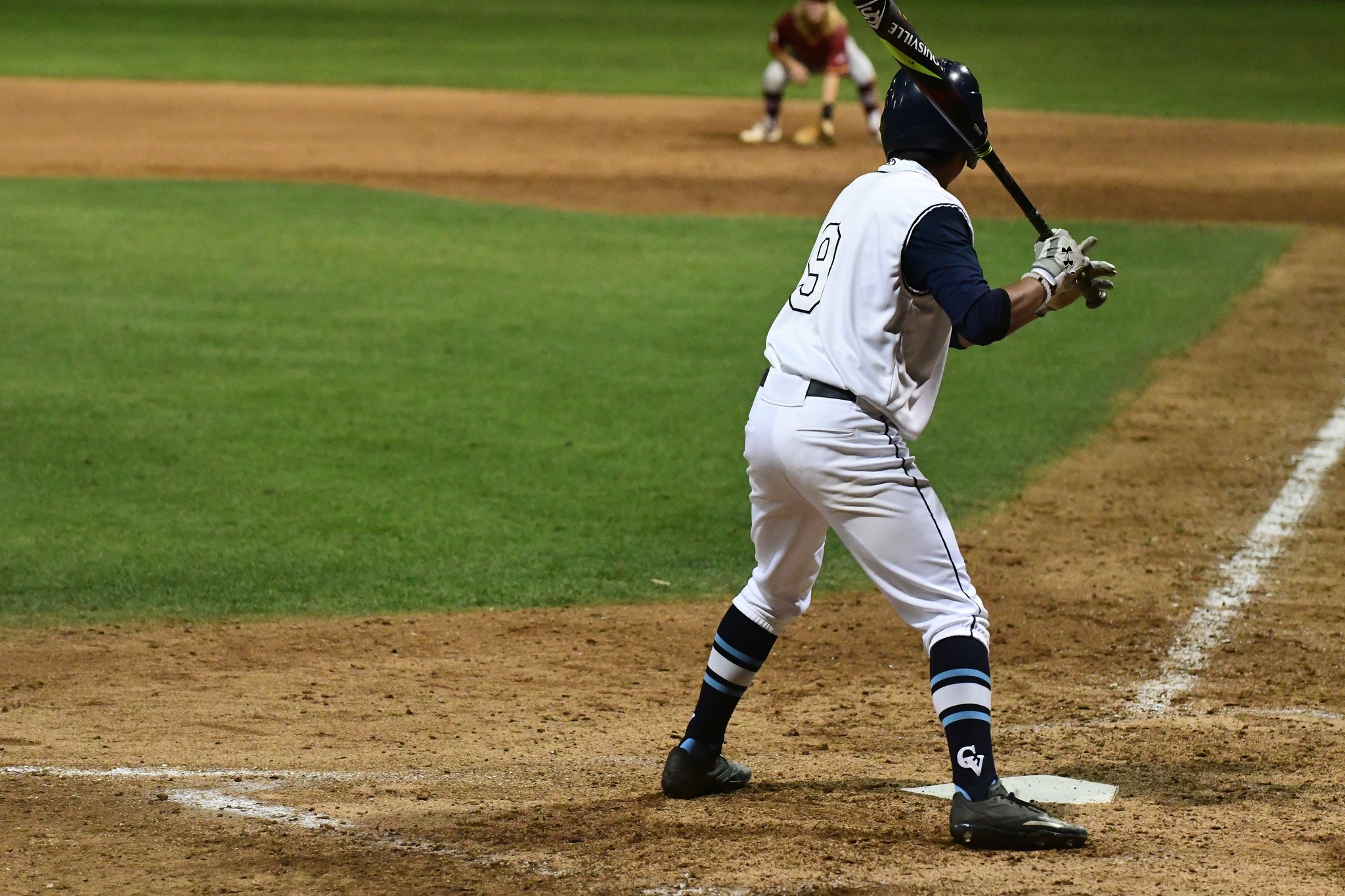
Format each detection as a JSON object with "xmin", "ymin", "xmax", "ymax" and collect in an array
[
  {"xmin": 662, "ymin": 744, "xmax": 752, "ymax": 797},
  {"xmin": 950, "ymin": 791, "xmax": 1090, "ymax": 850}
]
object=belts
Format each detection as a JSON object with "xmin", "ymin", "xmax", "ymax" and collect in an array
[{"xmin": 759, "ymin": 368, "xmax": 856, "ymax": 403}]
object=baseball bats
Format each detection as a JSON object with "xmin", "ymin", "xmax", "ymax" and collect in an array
[{"xmin": 848, "ymin": 0, "xmax": 1108, "ymax": 309}]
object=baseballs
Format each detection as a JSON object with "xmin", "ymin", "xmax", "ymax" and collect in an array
[{"xmin": 792, "ymin": 124, "xmax": 837, "ymax": 148}]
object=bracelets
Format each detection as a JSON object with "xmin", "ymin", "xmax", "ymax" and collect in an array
[{"xmin": 1021, "ymin": 273, "xmax": 1052, "ymax": 314}]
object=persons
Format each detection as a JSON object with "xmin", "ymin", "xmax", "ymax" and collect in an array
[
  {"xmin": 740, "ymin": 0, "xmax": 882, "ymax": 147},
  {"xmin": 661, "ymin": 58, "xmax": 1117, "ymax": 851}
]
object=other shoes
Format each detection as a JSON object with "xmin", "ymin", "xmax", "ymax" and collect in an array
[
  {"xmin": 740, "ymin": 119, "xmax": 770, "ymax": 143},
  {"xmin": 864, "ymin": 109, "xmax": 881, "ymax": 135}
]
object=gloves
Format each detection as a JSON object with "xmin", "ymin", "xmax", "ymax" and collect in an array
[
  {"xmin": 1020, "ymin": 229, "xmax": 1088, "ymax": 312},
  {"xmin": 1035, "ymin": 236, "xmax": 1117, "ymax": 318}
]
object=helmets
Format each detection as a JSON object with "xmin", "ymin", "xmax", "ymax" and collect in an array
[{"xmin": 880, "ymin": 57, "xmax": 985, "ymax": 155}]
